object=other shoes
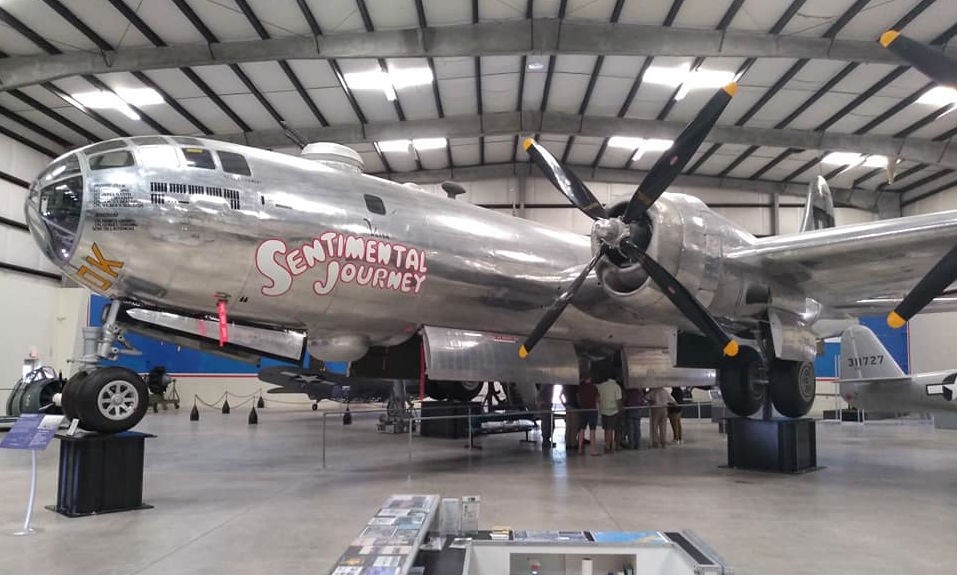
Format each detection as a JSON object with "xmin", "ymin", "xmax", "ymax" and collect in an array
[
  {"xmin": 566, "ymin": 437, "xmax": 684, "ymax": 457},
  {"xmin": 542, "ymin": 439, "xmax": 556, "ymax": 447}
]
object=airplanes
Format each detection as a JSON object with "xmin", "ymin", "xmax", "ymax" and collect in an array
[{"xmin": 23, "ymin": 31, "xmax": 957, "ymax": 436}]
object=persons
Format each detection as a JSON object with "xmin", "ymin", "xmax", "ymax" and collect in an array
[
  {"xmin": 560, "ymin": 369, "xmax": 598, "ymax": 449},
  {"xmin": 615, "ymin": 387, "xmax": 645, "ymax": 450},
  {"xmin": 535, "ymin": 383, "xmax": 556, "ymax": 450},
  {"xmin": 644, "ymin": 386, "xmax": 685, "ymax": 449},
  {"xmin": 596, "ymin": 370, "xmax": 623, "ymax": 452}
]
